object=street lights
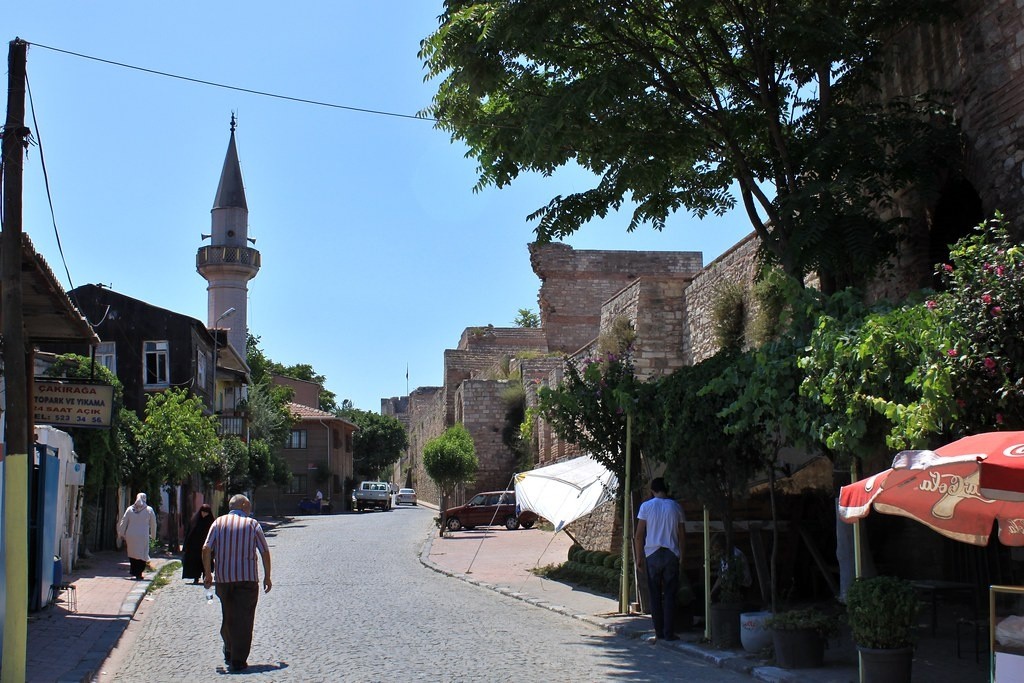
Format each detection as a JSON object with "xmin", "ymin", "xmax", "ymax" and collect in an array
[{"xmin": 210, "ymin": 307, "xmax": 237, "ymax": 431}]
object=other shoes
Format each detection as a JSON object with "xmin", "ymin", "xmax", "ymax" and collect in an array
[
  {"xmin": 658, "ymin": 633, "xmax": 681, "ymax": 642},
  {"xmin": 227, "ymin": 663, "xmax": 248, "ymax": 671},
  {"xmin": 193, "ymin": 579, "xmax": 199, "ymax": 584}
]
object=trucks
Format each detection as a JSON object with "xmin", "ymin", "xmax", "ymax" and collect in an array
[{"xmin": 355, "ymin": 481, "xmax": 393, "ymax": 512}]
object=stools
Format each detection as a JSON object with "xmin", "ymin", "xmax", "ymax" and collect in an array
[{"xmin": 45, "ymin": 583, "xmax": 79, "ymax": 616}]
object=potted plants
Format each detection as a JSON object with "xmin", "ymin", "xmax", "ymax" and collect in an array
[
  {"xmin": 760, "ymin": 604, "xmax": 834, "ymax": 670},
  {"xmin": 834, "ymin": 573, "xmax": 943, "ymax": 683},
  {"xmin": 704, "ymin": 539, "xmax": 756, "ymax": 651}
]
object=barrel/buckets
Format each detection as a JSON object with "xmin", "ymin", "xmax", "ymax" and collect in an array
[{"xmin": 53, "ymin": 555, "xmax": 64, "ymax": 599}]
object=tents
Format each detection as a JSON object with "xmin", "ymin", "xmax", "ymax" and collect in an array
[{"xmin": 464, "ymin": 449, "xmax": 643, "ymax": 594}]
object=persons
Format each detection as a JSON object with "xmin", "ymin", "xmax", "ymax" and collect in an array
[
  {"xmin": 315, "ymin": 489, "xmax": 323, "ymax": 514},
  {"xmin": 202, "ymin": 495, "xmax": 272, "ymax": 674},
  {"xmin": 635, "ymin": 476, "xmax": 686, "ymax": 641},
  {"xmin": 182, "ymin": 504, "xmax": 215, "ymax": 585},
  {"xmin": 119, "ymin": 492, "xmax": 157, "ymax": 581}
]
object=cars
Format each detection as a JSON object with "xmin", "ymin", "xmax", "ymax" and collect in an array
[
  {"xmin": 395, "ymin": 488, "xmax": 418, "ymax": 506},
  {"xmin": 390, "ymin": 483, "xmax": 399, "ymax": 494},
  {"xmin": 436, "ymin": 490, "xmax": 539, "ymax": 531}
]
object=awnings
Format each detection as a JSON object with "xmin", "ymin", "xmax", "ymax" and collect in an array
[{"xmin": 838, "ymin": 431, "xmax": 1024, "ymax": 547}]
